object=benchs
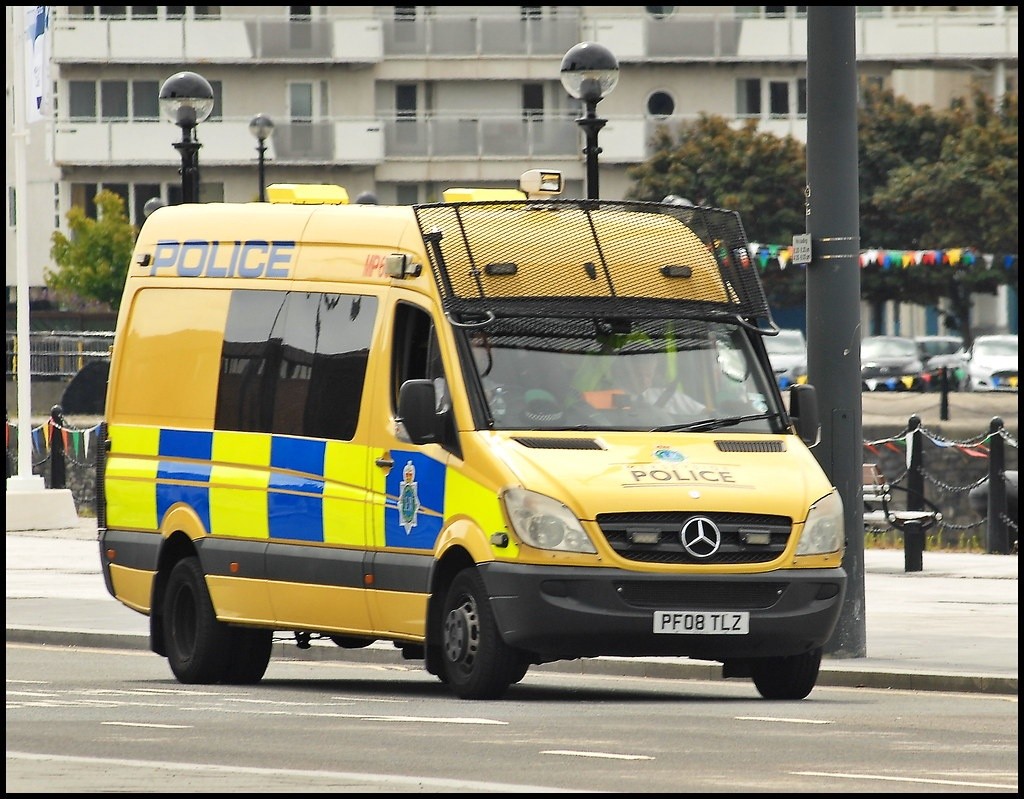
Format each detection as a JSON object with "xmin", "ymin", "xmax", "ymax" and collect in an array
[{"xmin": 862, "ymin": 464, "xmax": 947, "ymax": 573}]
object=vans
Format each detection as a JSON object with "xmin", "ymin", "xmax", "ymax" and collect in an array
[{"xmin": 99, "ymin": 168, "xmax": 849, "ymax": 706}]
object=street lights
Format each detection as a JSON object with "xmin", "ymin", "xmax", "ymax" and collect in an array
[
  {"xmin": 559, "ymin": 42, "xmax": 620, "ymax": 201},
  {"xmin": 246, "ymin": 114, "xmax": 277, "ymax": 202},
  {"xmin": 159, "ymin": 70, "xmax": 219, "ymax": 205}
]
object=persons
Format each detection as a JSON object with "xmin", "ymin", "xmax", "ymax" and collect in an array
[
  {"xmin": 616, "ymin": 347, "xmax": 720, "ymax": 422},
  {"xmin": 434, "ymin": 329, "xmax": 509, "ymax": 419}
]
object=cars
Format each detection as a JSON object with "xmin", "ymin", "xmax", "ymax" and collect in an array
[
  {"xmin": 968, "ymin": 334, "xmax": 1021, "ymax": 394},
  {"xmin": 858, "ymin": 331, "xmax": 919, "ymax": 394},
  {"xmin": 760, "ymin": 327, "xmax": 808, "ymax": 389},
  {"xmin": 915, "ymin": 334, "xmax": 969, "ymax": 391}
]
